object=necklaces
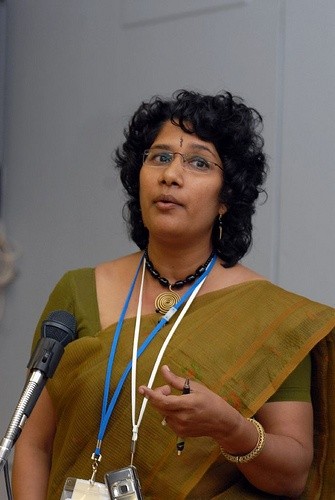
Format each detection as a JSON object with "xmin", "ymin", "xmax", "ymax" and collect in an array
[{"xmin": 145, "ymin": 249, "xmax": 215, "ymax": 315}]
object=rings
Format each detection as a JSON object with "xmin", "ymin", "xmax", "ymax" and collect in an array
[{"xmin": 161, "ymin": 416, "xmax": 167, "ymax": 427}]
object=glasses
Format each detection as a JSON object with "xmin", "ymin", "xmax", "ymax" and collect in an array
[{"xmin": 142, "ymin": 148, "xmax": 224, "ymax": 175}]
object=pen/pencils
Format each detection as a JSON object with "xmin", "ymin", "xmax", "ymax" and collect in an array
[{"xmin": 177, "ymin": 378, "xmax": 190, "ymax": 456}]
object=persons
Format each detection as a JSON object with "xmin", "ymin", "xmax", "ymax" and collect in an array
[{"xmin": 11, "ymin": 89, "xmax": 335, "ymax": 500}]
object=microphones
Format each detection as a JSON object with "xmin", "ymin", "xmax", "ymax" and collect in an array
[{"xmin": 0, "ymin": 310, "xmax": 78, "ymax": 472}]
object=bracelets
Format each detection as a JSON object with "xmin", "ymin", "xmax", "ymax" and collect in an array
[{"xmin": 220, "ymin": 417, "xmax": 265, "ymax": 464}]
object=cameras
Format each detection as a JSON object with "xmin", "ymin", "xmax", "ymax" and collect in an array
[{"xmin": 105, "ymin": 465, "xmax": 142, "ymax": 500}]
